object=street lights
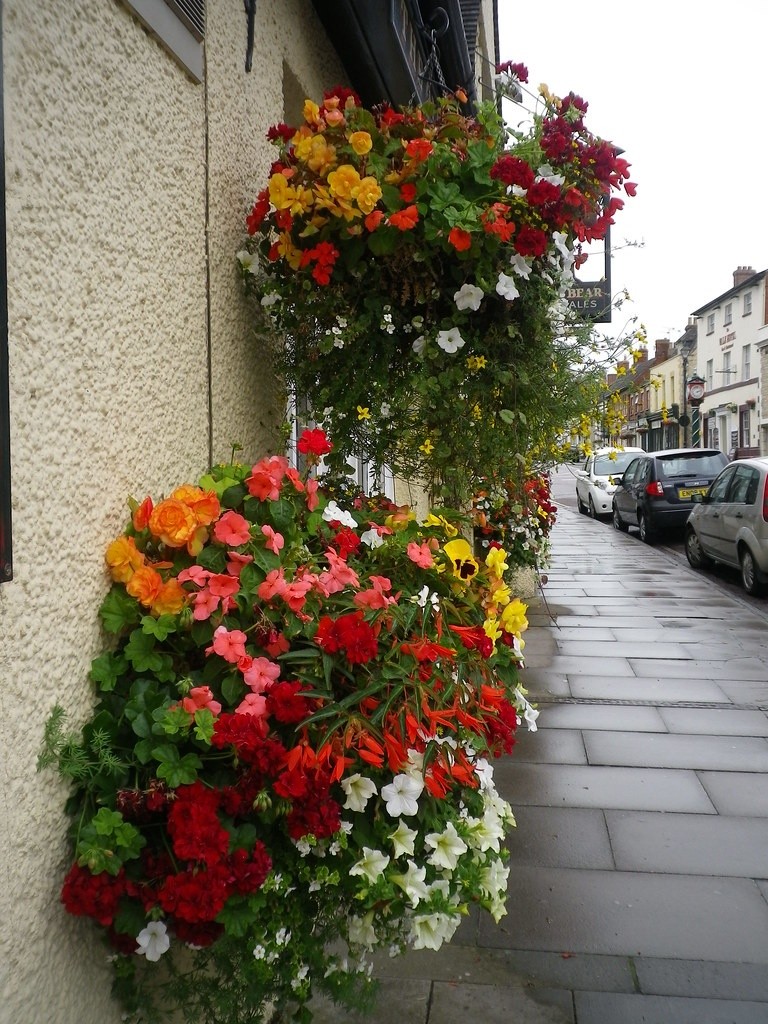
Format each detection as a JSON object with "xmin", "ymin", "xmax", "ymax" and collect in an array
[{"xmin": 680, "ymin": 342, "xmax": 693, "ymax": 448}]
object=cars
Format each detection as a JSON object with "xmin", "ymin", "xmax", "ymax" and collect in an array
[
  {"xmin": 611, "ymin": 447, "xmax": 732, "ymax": 546},
  {"xmin": 683, "ymin": 456, "xmax": 767, "ymax": 597},
  {"xmin": 576, "ymin": 446, "xmax": 647, "ymax": 521}
]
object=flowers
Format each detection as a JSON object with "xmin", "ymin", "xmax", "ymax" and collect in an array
[
  {"xmin": 473, "ymin": 466, "xmax": 554, "ymax": 566},
  {"xmin": 238, "ymin": 68, "xmax": 638, "ymax": 507},
  {"xmin": 36, "ymin": 427, "xmax": 539, "ymax": 1024}
]
